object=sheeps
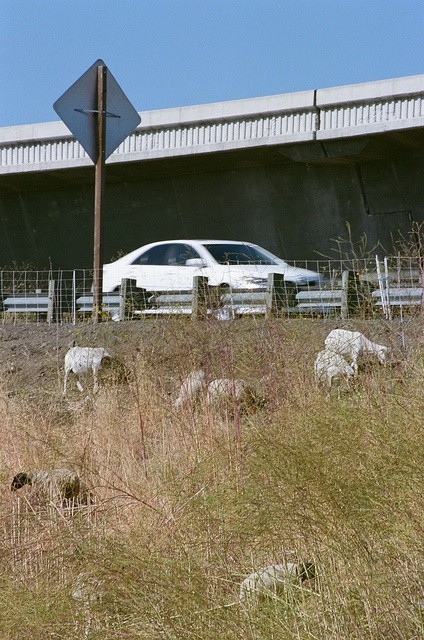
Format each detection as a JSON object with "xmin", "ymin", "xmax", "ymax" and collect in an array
[
  {"xmin": 234, "ymin": 559, "xmax": 320, "ymax": 613},
  {"xmin": 323, "ymin": 328, "xmax": 392, "ymax": 378},
  {"xmin": 172, "ymin": 369, "xmax": 207, "ymax": 417},
  {"xmin": 59, "ymin": 345, "xmax": 135, "ymax": 400},
  {"xmin": 7, "ymin": 466, "xmax": 92, "ymax": 522},
  {"xmin": 207, "ymin": 378, "xmax": 275, "ymax": 422},
  {"xmin": 313, "ymin": 350, "xmax": 360, "ymax": 409}
]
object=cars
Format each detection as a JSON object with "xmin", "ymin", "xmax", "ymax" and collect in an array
[{"xmin": 91, "ymin": 238, "xmax": 323, "ymax": 292}]
